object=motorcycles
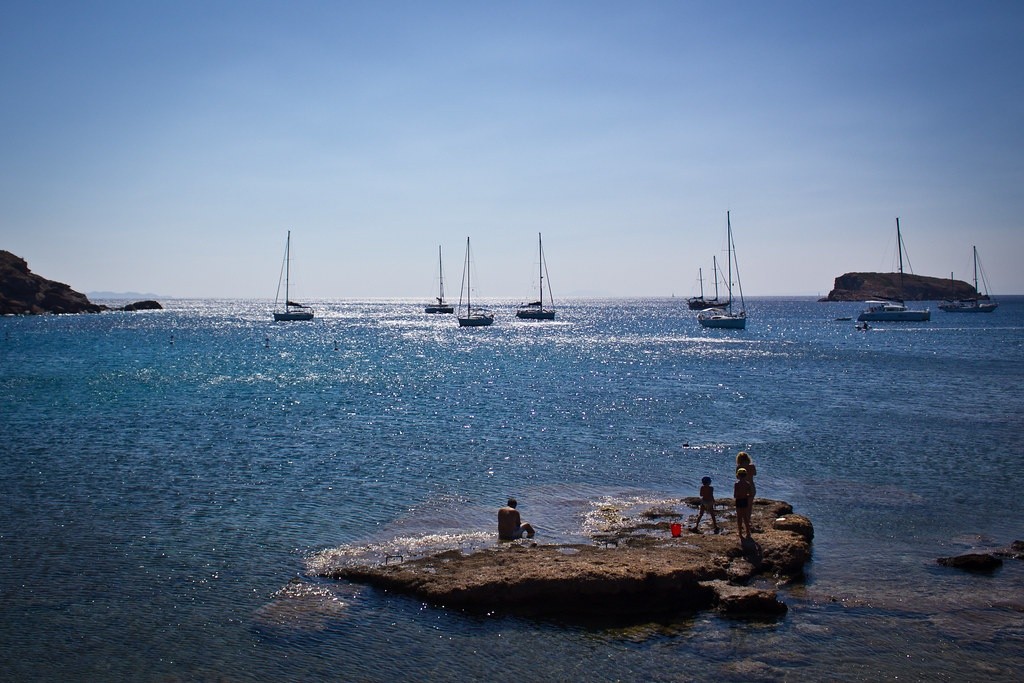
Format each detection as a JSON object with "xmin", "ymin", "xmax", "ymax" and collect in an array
[{"xmin": 855, "ymin": 324, "xmax": 873, "ymax": 332}]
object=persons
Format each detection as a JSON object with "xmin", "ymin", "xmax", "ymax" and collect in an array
[
  {"xmin": 693, "ymin": 476, "xmax": 719, "ymax": 531},
  {"xmin": 735, "ymin": 451, "xmax": 758, "ymax": 520},
  {"xmin": 498, "ymin": 498, "xmax": 532, "ymax": 539},
  {"xmin": 734, "ymin": 468, "xmax": 753, "ymax": 538}
]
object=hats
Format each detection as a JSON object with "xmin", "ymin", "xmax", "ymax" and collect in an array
[{"xmin": 737, "ymin": 467, "xmax": 747, "ymax": 475}]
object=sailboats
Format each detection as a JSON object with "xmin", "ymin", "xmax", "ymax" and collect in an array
[
  {"xmin": 856, "ymin": 217, "xmax": 932, "ymax": 322},
  {"xmin": 696, "ymin": 210, "xmax": 747, "ymax": 329},
  {"xmin": 272, "ymin": 230, "xmax": 315, "ymax": 322},
  {"xmin": 685, "ymin": 256, "xmax": 731, "ymax": 309},
  {"xmin": 424, "ymin": 245, "xmax": 453, "ymax": 314},
  {"xmin": 457, "ymin": 236, "xmax": 494, "ymax": 326},
  {"xmin": 938, "ymin": 245, "xmax": 999, "ymax": 313},
  {"xmin": 516, "ymin": 232, "xmax": 556, "ymax": 320}
]
